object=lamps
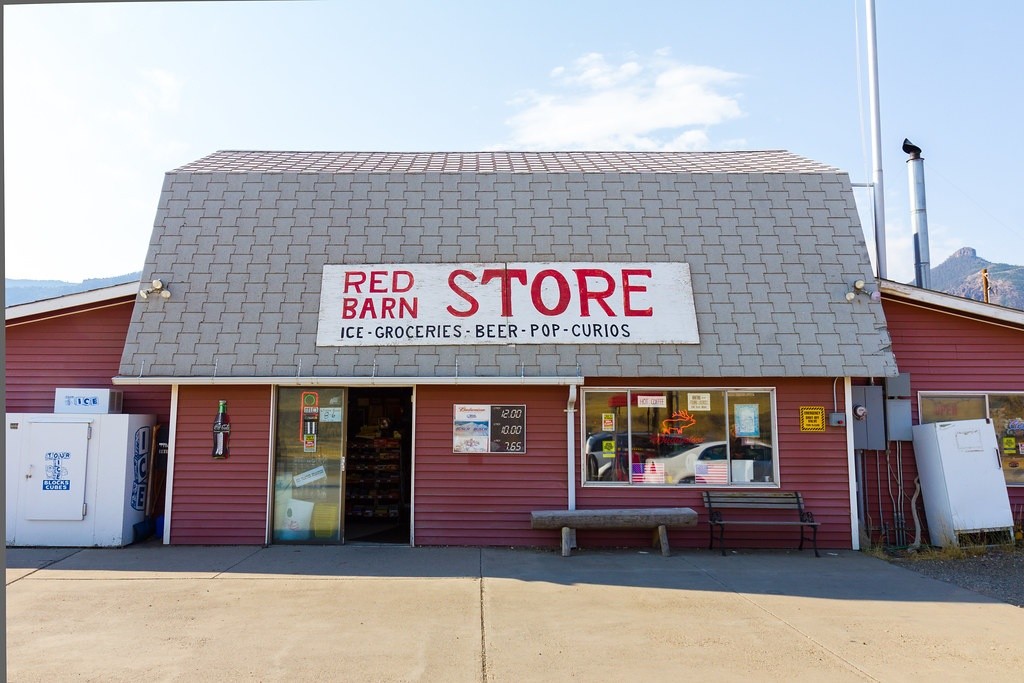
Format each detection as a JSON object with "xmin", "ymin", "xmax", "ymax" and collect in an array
[
  {"xmin": 140, "ymin": 280, "xmax": 172, "ymax": 299},
  {"xmin": 846, "ymin": 280, "xmax": 881, "ymax": 303}
]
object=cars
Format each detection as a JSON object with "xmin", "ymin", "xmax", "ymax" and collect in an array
[{"xmin": 642, "ymin": 438, "xmax": 773, "ymax": 484}]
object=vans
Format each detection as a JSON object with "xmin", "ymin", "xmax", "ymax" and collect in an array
[{"xmin": 585, "ymin": 434, "xmax": 699, "ymax": 481}]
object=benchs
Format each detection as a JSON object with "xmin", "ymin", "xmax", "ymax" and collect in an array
[
  {"xmin": 702, "ymin": 490, "xmax": 822, "ymax": 558},
  {"xmin": 531, "ymin": 508, "xmax": 699, "ymax": 557}
]
